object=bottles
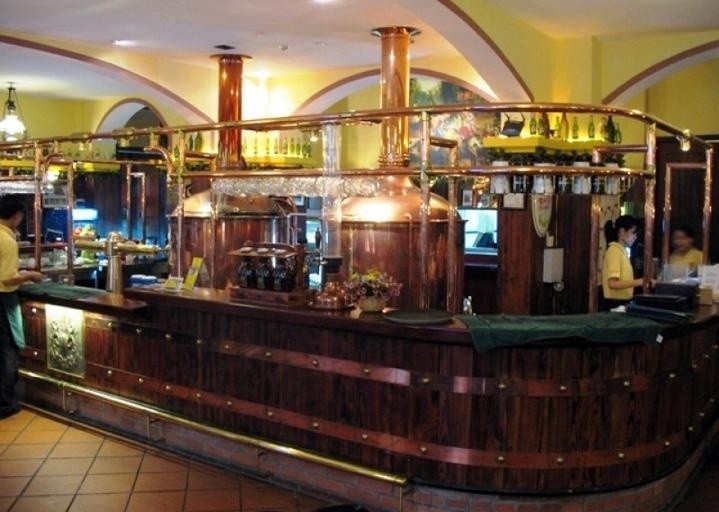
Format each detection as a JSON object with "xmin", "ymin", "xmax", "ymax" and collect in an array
[
  {"xmin": 184, "ymin": 131, "xmax": 314, "ymax": 159},
  {"xmin": 528, "ymin": 111, "xmax": 622, "ymax": 145}
]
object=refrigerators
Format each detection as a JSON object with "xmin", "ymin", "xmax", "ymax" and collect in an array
[{"xmin": 42, "ymin": 208, "xmax": 98, "ymax": 243}]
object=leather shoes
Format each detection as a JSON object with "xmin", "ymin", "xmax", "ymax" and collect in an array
[{"xmin": 0, "ymin": 405, "xmax": 21, "ymax": 419}]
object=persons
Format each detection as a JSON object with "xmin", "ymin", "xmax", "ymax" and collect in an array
[
  {"xmin": 602, "ymin": 215, "xmax": 657, "ymax": 312},
  {"xmin": 0, "ymin": 193, "xmax": 45, "ymax": 420},
  {"xmin": 668, "ymin": 228, "xmax": 710, "ymax": 277}
]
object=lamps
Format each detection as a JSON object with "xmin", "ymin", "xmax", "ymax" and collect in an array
[{"xmin": 0, "ymin": 81, "xmax": 26, "ymax": 142}]
